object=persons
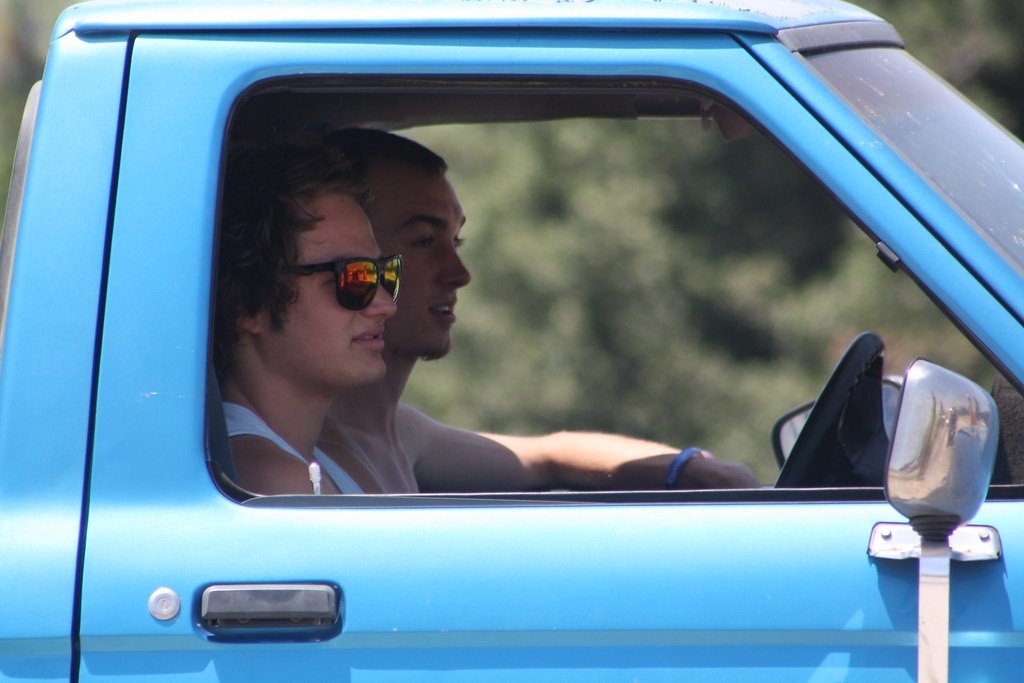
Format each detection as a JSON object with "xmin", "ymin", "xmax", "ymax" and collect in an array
[
  {"xmin": 215, "ymin": 136, "xmax": 400, "ymax": 498},
  {"xmin": 316, "ymin": 126, "xmax": 761, "ymax": 498}
]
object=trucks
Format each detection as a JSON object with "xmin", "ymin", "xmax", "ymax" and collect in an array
[{"xmin": 0, "ymin": 0, "xmax": 1024, "ymax": 683}]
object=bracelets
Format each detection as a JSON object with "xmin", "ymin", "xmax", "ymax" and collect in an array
[{"xmin": 664, "ymin": 446, "xmax": 709, "ymax": 483}]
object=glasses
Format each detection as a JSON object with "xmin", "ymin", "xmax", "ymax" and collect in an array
[{"xmin": 292, "ymin": 253, "xmax": 404, "ymax": 310}]
